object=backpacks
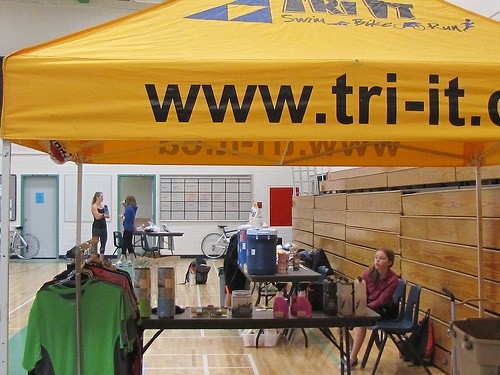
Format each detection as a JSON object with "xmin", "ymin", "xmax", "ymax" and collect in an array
[
  {"xmin": 403, "ymin": 308, "xmax": 434, "ymax": 367},
  {"xmin": 185, "ymin": 256, "xmax": 210, "ymax": 284},
  {"xmin": 290, "ymin": 247, "xmax": 335, "ymax": 311}
]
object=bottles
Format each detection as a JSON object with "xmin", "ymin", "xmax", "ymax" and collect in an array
[{"xmin": 323, "ymin": 276, "xmax": 368, "ymax": 315}]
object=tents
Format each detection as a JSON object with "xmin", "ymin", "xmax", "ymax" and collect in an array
[{"xmin": 0, "ymin": 0, "xmax": 500, "ymax": 375}]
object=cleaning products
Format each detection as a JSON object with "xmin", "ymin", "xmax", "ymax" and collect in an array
[
  {"xmin": 273, "ymin": 282, "xmax": 290, "ymax": 318},
  {"xmin": 292, "ymin": 281, "xmax": 312, "ymax": 317}
]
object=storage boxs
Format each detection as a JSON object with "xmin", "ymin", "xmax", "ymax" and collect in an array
[
  {"xmin": 252, "ymin": 305, "xmax": 274, "ymax": 318},
  {"xmin": 240, "ymin": 328, "xmax": 285, "ymax": 348}
]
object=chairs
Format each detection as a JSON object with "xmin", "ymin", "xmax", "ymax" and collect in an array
[
  {"xmin": 364, "ymin": 279, "xmax": 434, "ymax": 375},
  {"xmin": 110, "ymin": 231, "xmax": 158, "ymax": 263}
]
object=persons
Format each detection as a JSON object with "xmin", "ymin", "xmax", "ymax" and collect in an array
[
  {"xmin": 91, "ymin": 192, "xmax": 110, "ymax": 261},
  {"xmin": 224, "ymin": 234, "xmax": 246, "ymax": 307},
  {"xmin": 337, "ymin": 248, "xmax": 399, "ymax": 371},
  {"xmin": 116, "ymin": 195, "xmax": 138, "ymax": 264}
]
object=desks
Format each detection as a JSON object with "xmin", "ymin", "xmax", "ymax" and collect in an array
[
  {"xmin": 239, "ymin": 259, "xmax": 322, "ymax": 348},
  {"xmin": 132, "ymin": 230, "xmax": 184, "ymax": 256},
  {"xmin": 126, "ymin": 305, "xmax": 381, "ymax": 375}
]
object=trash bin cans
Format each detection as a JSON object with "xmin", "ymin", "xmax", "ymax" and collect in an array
[
  {"xmin": 217, "ymin": 266, "xmax": 226, "ymax": 308},
  {"xmin": 451, "ymin": 316, "xmax": 496, "ymax": 375}
]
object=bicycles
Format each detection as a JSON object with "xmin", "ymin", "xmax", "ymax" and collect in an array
[
  {"xmin": 201, "ymin": 225, "xmax": 239, "ymax": 259},
  {"xmin": 10, "ymin": 225, "xmax": 40, "ymax": 260}
]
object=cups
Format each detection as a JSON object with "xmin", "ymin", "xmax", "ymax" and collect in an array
[{"xmin": 293, "ymin": 254, "xmax": 301, "ymax": 271}]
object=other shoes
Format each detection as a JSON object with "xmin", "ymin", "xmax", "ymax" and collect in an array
[
  {"xmin": 114, "ymin": 259, "xmax": 133, "ymax": 267},
  {"xmin": 339, "ymin": 357, "xmax": 358, "ymax": 372}
]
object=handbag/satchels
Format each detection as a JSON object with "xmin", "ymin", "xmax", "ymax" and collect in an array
[{"xmin": 336, "ymin": 277, "xmax": 367, "ymax": 314}]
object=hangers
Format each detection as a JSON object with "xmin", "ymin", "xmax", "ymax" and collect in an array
[{"xmin": 53, "ymin": 237, "xmax": 106, "ymax": 288}]
